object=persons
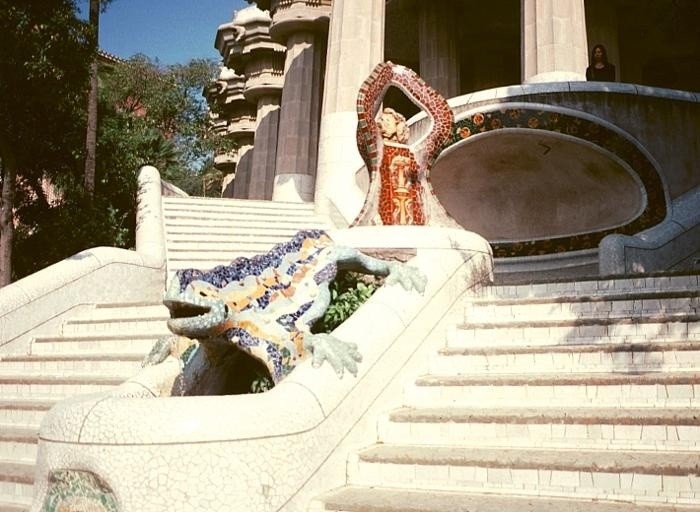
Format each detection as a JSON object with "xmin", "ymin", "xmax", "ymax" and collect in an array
[{"xmin": 586, "ymin": 44, "xmax": 615, "ymax": 82}]
